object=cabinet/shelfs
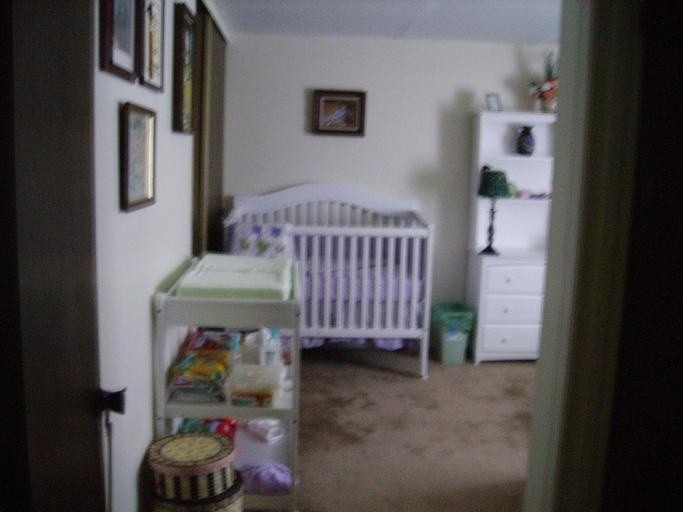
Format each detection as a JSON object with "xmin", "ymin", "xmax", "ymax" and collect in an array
[
  {"xmin": 466, "ymin": 110, "xmax": 558, "ymax": 368},
  {"xmin": 151, "ymin": 252, "xmax": 303, "ymax": 511}
]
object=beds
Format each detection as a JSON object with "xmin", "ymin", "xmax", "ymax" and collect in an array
[{"xmin": 220, "ymin": 179, "xmax": 436, "ymax": 378}]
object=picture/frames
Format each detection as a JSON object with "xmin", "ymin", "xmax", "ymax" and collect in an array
[
  {"xmin": 313, "ymin": 89, "xmax": 366, "ymax": 137},
  {"xmin": 99, "ymin": 0, "xmax": 198, "ymax": 213}
]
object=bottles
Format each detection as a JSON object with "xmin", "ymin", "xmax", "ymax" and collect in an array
[{"xmin": 516, "ymin": 126, "xmax": 535, "ymax": 156}]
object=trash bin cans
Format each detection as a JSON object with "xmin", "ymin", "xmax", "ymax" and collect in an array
[{"xmin": 432, "ymin": 301, "xmax": 475, "ymax": 365}]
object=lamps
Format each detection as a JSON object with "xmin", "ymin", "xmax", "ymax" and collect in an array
[{"xmin": 477, "ymin": 170, "xmax": 512, "ymax": 256}]
